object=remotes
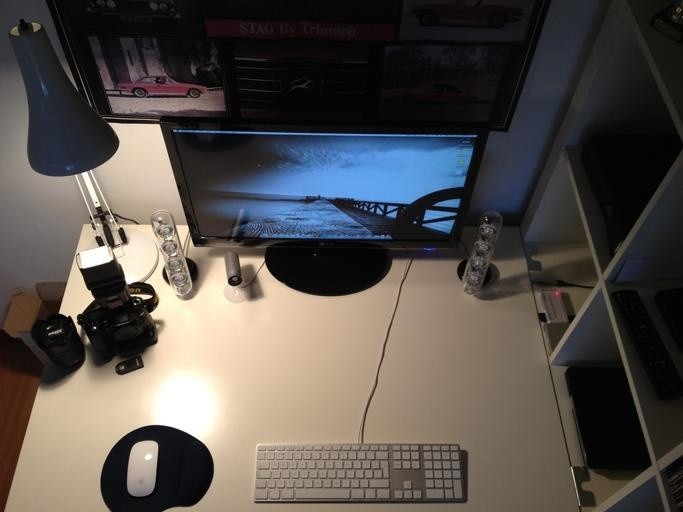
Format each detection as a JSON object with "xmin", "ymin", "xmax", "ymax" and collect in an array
[{"xmin": 610, "ymin": 290, "xmax": 683, "ymax": 400}]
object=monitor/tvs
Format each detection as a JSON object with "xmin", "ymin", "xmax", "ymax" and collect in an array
[{"xmin": 160, "ymin": 114, "xmax": 490, "ymax": 296}]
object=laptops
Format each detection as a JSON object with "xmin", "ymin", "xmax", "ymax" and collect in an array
[{"xmin": 565, "ymin": 366, "xmax": 653, "ymax": 470}]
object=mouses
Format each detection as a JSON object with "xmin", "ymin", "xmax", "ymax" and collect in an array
[{"xmin": 127, "ymin": 440, "xmax": 159, "ymax": 498}]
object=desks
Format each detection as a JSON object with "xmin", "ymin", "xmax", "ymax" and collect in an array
[{"xmin": 0, "ymin": 223, "xmax": 601, "ymax": 512}]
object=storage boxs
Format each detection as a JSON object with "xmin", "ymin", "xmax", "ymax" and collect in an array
[{"xmin": 0, "ymin": 281, "xmax": 66, "ymax": 366}]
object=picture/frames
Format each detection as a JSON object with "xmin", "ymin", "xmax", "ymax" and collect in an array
[{"xmin": 46, "ymin": 0, "xmax": 552, "ymax": 134}]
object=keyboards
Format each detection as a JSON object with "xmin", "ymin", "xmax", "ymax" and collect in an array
[{"xmin": 254, "ymin": 442, "xmax": 467, "ymax": 503}]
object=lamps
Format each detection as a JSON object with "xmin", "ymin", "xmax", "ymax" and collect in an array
[{"xmin": 8, "ymin": 18, "xmax": 159, "ymax": 285}]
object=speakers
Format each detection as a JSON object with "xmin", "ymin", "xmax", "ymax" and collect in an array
[
  {"xmin": 151, "ymin": 210, "xmax": 198, "ymax": 296},
  {"xmin": 456, "ymin": 210, "xmax": 502, "ymax": 296}
]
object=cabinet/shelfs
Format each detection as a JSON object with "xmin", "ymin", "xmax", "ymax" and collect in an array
[{"xmin": 520, "ymin": 0, "xmax": 683, "ymax": 512}]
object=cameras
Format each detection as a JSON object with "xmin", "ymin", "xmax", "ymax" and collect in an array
[{"xmin": 76, "ymin": 244, "xmax": 161, "ymax": 364}]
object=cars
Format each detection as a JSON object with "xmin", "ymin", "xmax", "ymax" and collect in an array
[{"xmin": 115, "ymin": 74, "xmax": 208, "ymax": 100}]
